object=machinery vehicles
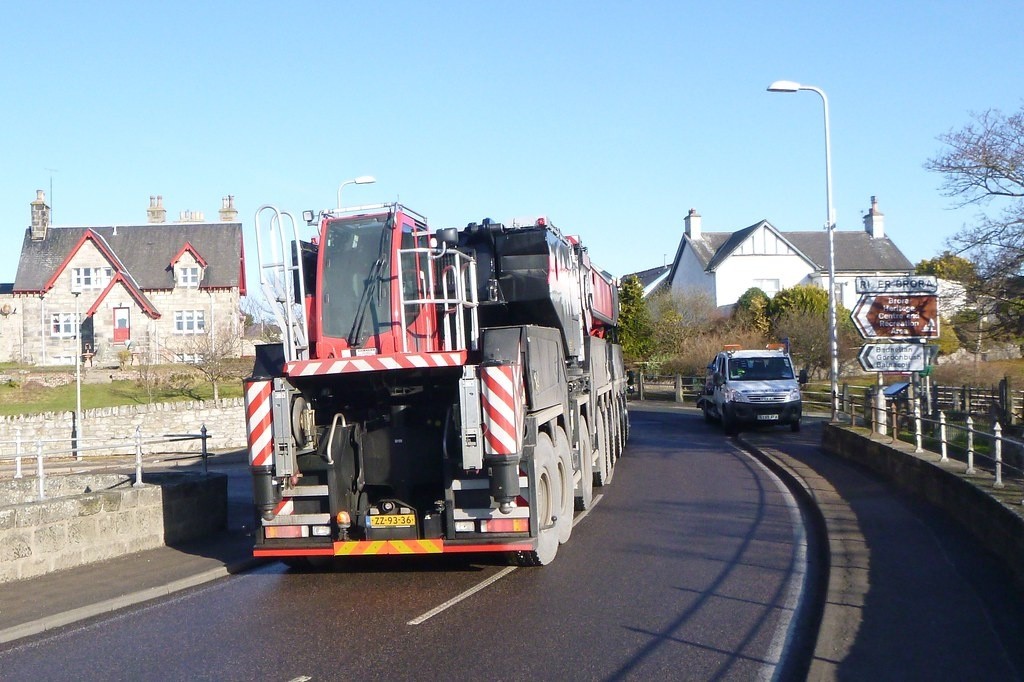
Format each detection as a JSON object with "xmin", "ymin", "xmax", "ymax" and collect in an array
[{"xmin": 241, "ymin": 202, "xmax": 634, "ymax": 567}]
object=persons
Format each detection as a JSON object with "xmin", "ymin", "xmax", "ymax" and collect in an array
[{"xmin": 731, "ymin": 361, "xmax": 745, "ymax": 376}]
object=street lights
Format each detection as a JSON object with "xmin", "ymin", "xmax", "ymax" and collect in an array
[
  {"xmin": 337, "ymin": 175, "xmax": 376, "ymax": 217},
  {"xmin": 767, "ymin": 80, "xmax": 839, "ymax": 423},
  {"xmin": 71, "ymin": 287, "xmax": 83, "ymax": 461}
]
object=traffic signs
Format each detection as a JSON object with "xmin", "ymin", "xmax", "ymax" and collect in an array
[
  {"xmin": 851, "ymin": 293, "xmax": 940, "ymax": 340},
  {"xmin": 857, "ymin": 343, "xmax": 924, "ymax": 371}
]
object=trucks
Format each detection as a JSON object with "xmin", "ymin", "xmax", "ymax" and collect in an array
[{"xmin": 696, "ymin": 343, "xmax": 807, "ymax": 435}]
